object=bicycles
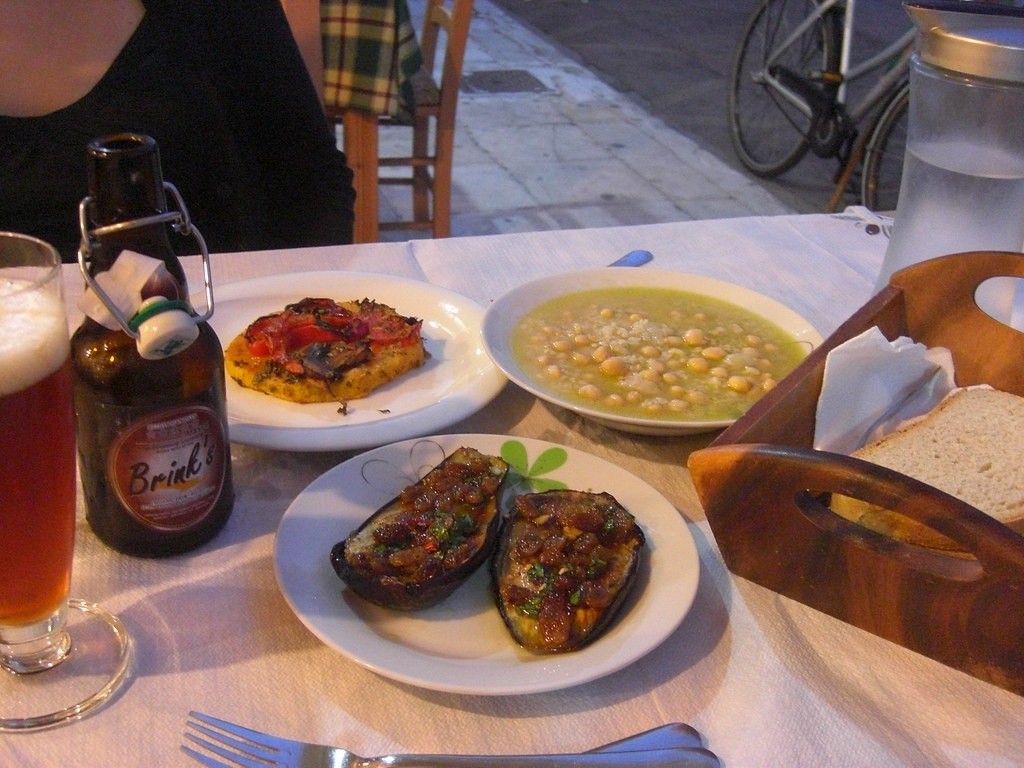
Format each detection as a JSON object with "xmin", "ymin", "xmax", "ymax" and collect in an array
[{"xmin": 728, "ymin": 1, "xmax": 912, "ymax": 207}]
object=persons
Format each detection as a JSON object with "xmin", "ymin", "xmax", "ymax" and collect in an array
[{"xmin": 1, "ymin": 1, "xmax": 358, "ymax": 266}]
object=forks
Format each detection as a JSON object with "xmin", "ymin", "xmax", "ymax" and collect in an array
[{"xmin": 178, "ymin": 708, "xmax": 723, "ymax": 767}]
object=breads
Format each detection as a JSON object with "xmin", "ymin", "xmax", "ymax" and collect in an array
[{"xmin": 826, "ymin": 388, "xmax": 1023, "ymax": 551}]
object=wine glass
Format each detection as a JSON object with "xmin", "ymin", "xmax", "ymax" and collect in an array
[{"xmin": 0, "ymin": 229, "xmax": 133, "ymax": 734}]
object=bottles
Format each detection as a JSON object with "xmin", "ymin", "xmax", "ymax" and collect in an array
[{"xmin": 68, "ymin": 129, "xmax": 235, "ymax": 568}]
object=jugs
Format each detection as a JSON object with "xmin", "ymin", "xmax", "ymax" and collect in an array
[{"xmin": 867, "ymin": 1, "xmax": 1020, "ymax": 305}]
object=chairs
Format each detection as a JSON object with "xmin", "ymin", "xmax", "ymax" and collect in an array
[{"xmin": 330, "ymin": 0, "xmax": 479, "ymax": 244}]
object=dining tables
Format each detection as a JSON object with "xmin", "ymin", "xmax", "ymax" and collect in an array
[{"xmin": 0, "ymin": 197, "xmax": 1024, "ymax": 767}]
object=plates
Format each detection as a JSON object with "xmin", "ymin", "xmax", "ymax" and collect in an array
[
  {"xmin": 482, "ymin": 264, "xmax": 827, "ymax": 437},
  {"xmin": 270, "ymin": 428, "xmax": 704, "ymax": 698},
  {"xmin": 183, "ymin": 267, "xmax": 511, "ymax": 456}
]
